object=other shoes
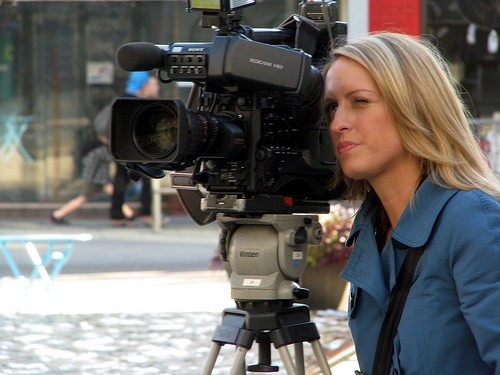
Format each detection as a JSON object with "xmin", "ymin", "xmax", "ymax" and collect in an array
[{"xmin": 47, "ymin": 214, "xmax": 69, "ymax": 225}]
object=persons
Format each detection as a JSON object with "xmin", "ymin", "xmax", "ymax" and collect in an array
[
  {"xmin": 50, "ymin": 72, "xmax": 172, "ymax": 229},
  {"xmin": 323, "ymin": 33, "xmax": 500, "ymax": 375}
]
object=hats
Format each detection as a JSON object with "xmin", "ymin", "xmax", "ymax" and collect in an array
[{"xmin": 126, "ymin": 71, "xmax": 147, "ymax": 96}]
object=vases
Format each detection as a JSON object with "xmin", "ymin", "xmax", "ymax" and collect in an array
[{"xmin": 300, "ymin": 261, "xmax": 347, "ymax": 311}]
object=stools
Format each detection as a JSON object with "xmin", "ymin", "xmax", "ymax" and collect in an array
[
  {"xmin": 0, "ymin": 116, "xmax": 36, "ymax": 161},
  {"xmin": 0, "ymin": 233, "xmax": 93, "ymax": 278}
]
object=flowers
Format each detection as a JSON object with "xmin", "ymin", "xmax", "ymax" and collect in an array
[{"xmin": 292, "ymin": 205, "xmax": 359, "ymax": 267}]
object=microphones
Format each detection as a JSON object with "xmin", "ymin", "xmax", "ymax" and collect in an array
[{"xmin": 116, "ymin": 42, "xmax": 166, "ymax": 72}]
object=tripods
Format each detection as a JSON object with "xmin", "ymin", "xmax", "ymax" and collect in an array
[{"xmin": 193, "ymin": 198, "xmax": 335, "ymax": 375}]
